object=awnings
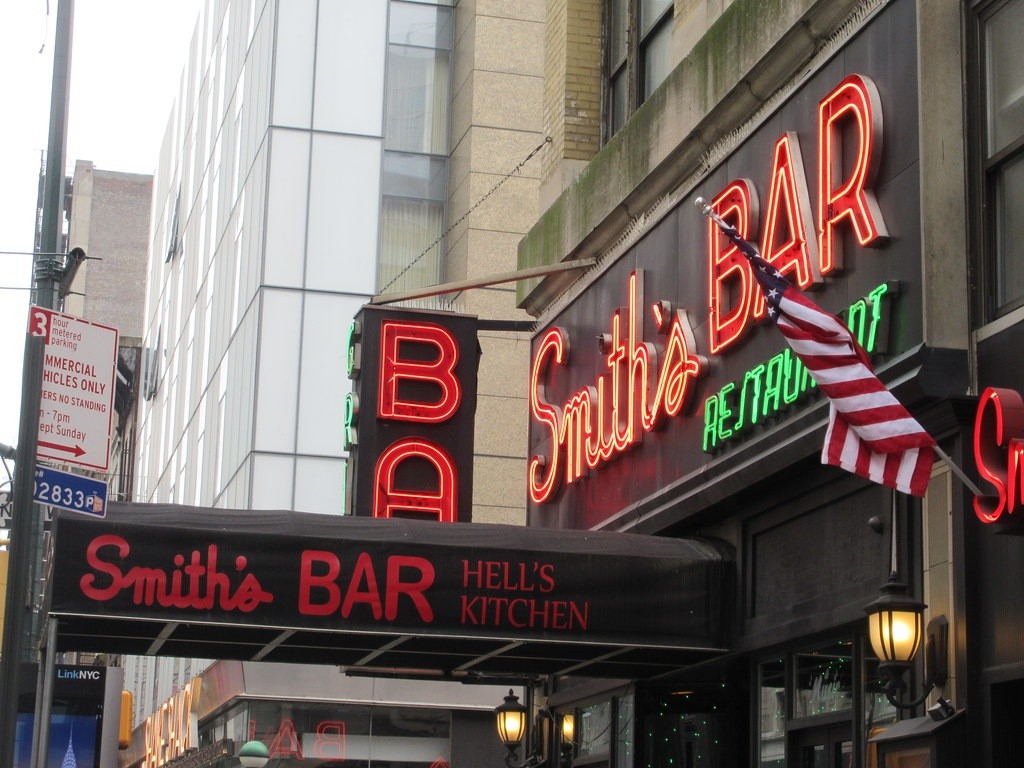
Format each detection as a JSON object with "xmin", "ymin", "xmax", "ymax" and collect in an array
[{"xmin": 31, "ymin": 502, "xmax": 734, "ymax": 768}]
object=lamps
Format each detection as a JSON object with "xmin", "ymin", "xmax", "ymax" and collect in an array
[
  {"xmin": 494, "ymin": 688, "xmax": 535, "ymax": 768},
  {"xmin": 862, "ymin": 568, "xmax": 948, "ymax": 710},
  {"xmin": 560, "ymin": 713, "xmax": 575, "ymax": 768}
]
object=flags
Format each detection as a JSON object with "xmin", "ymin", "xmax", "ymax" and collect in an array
[{"xmin": 723, "ymin": 227, "xmax": 936, "ymax": 496}]
